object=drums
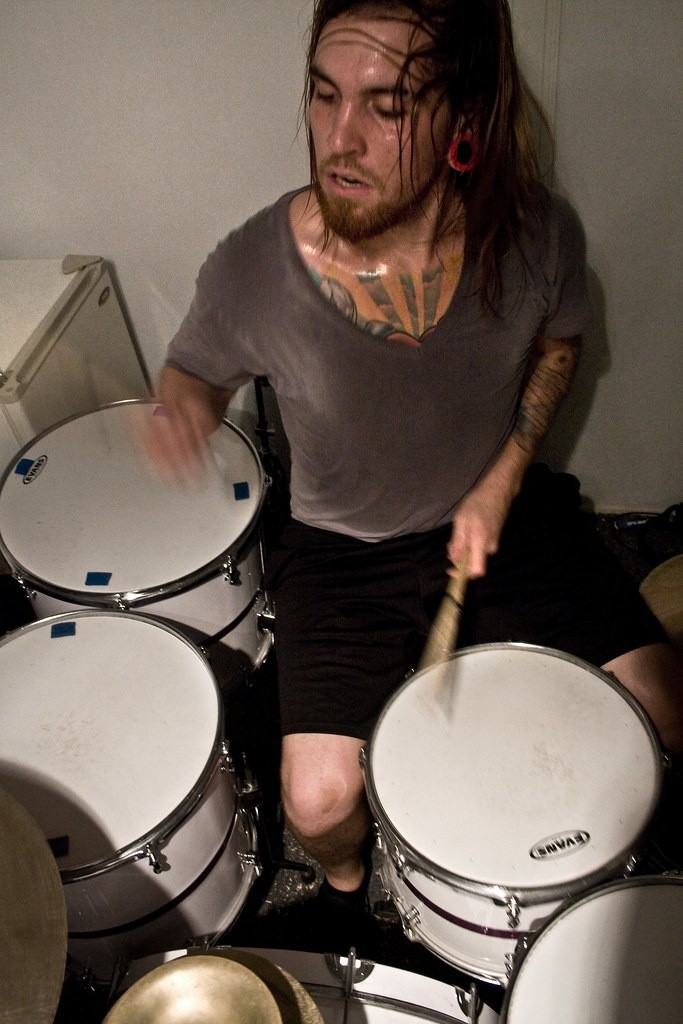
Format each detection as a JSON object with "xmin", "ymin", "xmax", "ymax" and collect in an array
[
  {"xmin": 360, "ymin": 644, "xmax": 661, "ymax": 989},
  {"xmin": 0, "ymin": 400, "xmax": 277, "ymax": 686},
  {"xmin": 0, "ymin": 606, "xmax": 262, "ymax": 993},
  {"xmin": 497, "ymin": 871, "xmax": 683, "ymax": 1024},
  {"xmin": 120, "ymin": 946, "xmax": 502, "ymax": 1024}
]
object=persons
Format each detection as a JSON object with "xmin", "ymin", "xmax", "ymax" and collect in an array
[{"xmin": 142, "ymin": 0, "xmax": 683, "ymax": 957}]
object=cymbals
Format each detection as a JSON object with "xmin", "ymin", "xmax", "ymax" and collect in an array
[{"xmin": 1, "ymin": 777, "xmax": 326, "ymax": 1024}]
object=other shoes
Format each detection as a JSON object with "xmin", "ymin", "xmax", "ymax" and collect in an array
[{"xmin": 308, "ymin": 862, "xmax": 374, "ymax": 955}]
